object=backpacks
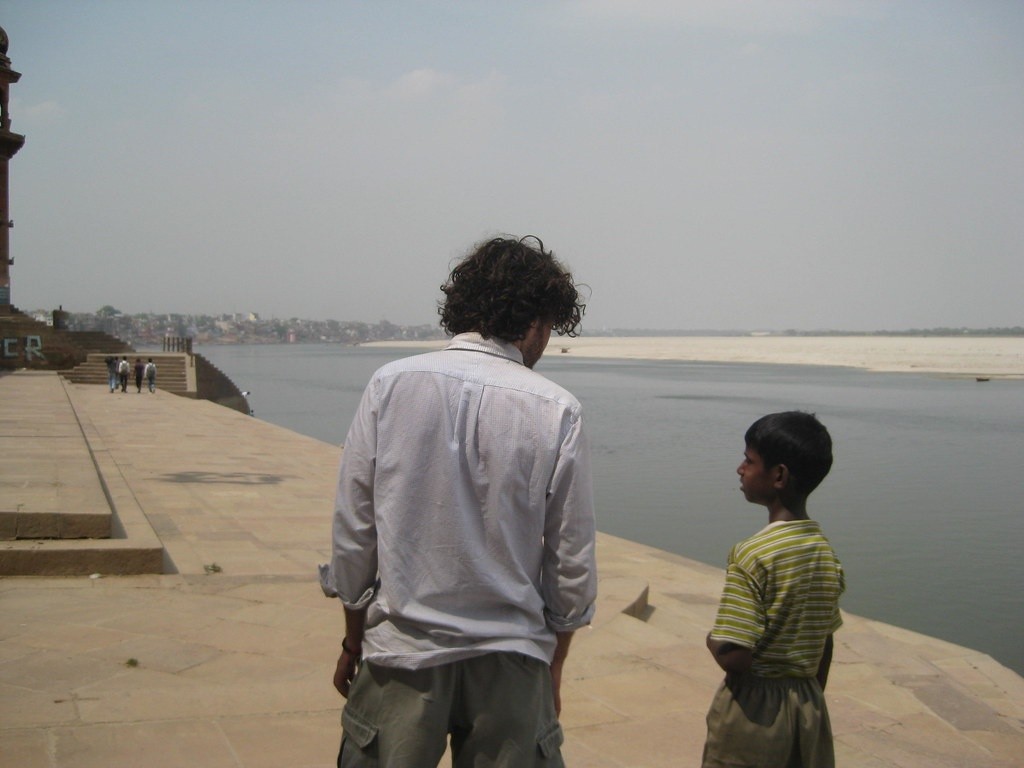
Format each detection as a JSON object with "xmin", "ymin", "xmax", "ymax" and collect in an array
[
  {"xmin": 120, "ymin": 361, "xmax": 128, "ymax": 376},
  {"xmin": 147, "ymin": 364, "xmax": 155, "ymax": 379}
]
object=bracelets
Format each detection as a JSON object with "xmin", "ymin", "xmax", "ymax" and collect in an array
[{"xmin": 341, "ymin": 637, "xmax": 352, "ymax": 657}]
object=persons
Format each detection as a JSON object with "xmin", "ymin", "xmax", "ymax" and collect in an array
[
  {"xmin": 700, "ymin": 411, "xmax": 846, "ymax": 768},
  {"xmin": 133, "ymin": 358, "xmax": 144, "ymax": 393},
  {"xmin": 242, "ymin": 391, "xmax": 250, "ymax": 398},
  {"xmin": 144, "ymin": 358, "xmax": 157, "ymax": 393},
  {"xmin": 107, "ymin": 355, "xmax": 129, "ymax": 393},
  {"xmin": 319, "ymin": 236, "xmax": 599, "ymax": 768}
]
